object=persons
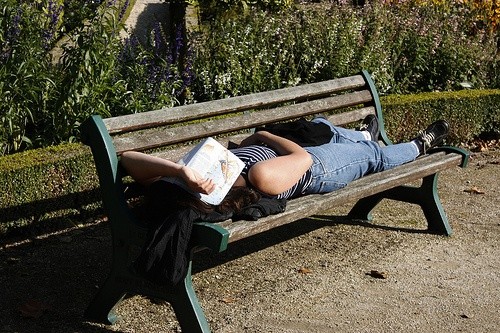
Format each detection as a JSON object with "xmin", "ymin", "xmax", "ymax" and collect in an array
[{"xmin": 120, "ymin": 114, "xmax": 450, "ymax": 212}]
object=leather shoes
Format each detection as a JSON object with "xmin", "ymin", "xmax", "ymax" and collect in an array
[
  {"xmin": 360, "ymin": 114, "xmax": 379, "ymax": 143},
  {"xmin": 414, "ymin": 120, "xmax": 450, "ymax": 154}
]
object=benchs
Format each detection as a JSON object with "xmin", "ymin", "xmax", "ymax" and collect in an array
[{"xmin": 84, "ymin": 67, "xmax": 471, "ymax": 333}]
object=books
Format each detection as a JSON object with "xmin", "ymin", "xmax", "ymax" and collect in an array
[{"xmin": 159, "ymin": 136, "xmax": 246, "ymax": 206}]
